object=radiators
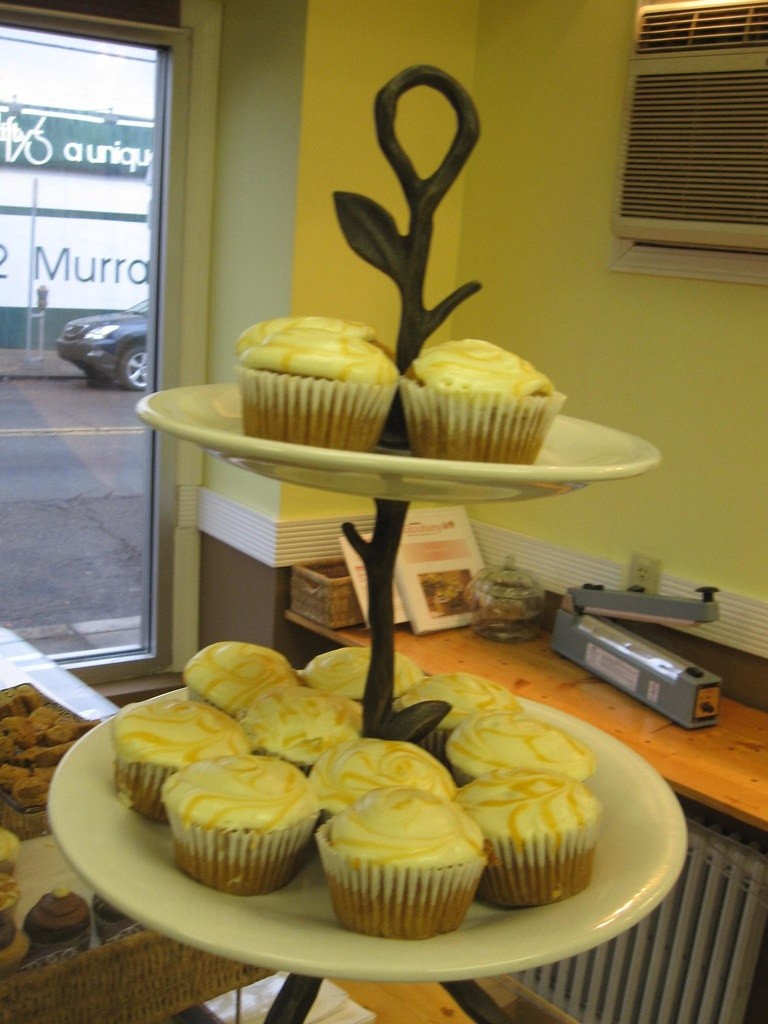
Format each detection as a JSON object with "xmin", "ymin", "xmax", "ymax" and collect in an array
[{"xmin": 503, "ymin": 795, "xmax": 768, "ymax": 1024}]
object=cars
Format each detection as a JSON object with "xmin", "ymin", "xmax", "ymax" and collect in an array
[{"xmin": 53, "ymin": 299, "xmax": 146, "ymax": 391}]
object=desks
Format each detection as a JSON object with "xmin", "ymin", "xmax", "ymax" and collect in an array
[
  {"xmin": 280, "ymin": 608, "xmax": 768, "ymax": 833},
  {"xmin": 2, "ymin": 628, "xmax": 582, "ymax": 1024}
]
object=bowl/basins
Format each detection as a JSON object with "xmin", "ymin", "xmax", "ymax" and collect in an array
[{"xmin": 460, "ymin": 563, "xmax": 547, "ymax": 643}]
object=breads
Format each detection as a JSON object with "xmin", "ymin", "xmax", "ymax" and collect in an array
[{"xmin": 1, "ymin": 685, "xmax": 103, "ymax": 807}]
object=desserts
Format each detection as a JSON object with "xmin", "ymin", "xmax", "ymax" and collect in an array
[
  {"xmin": 233, "ymin": 317, "xmax": 568, "ymax": 464},
  {"xmin": 111, "ymin": 641, "xmax": 603, "ymax": 943},
  {"xmin": 1, "ymin": 828, "xmax": 135, "ymax": 971}
]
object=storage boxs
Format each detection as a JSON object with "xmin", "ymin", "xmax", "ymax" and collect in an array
[
  {"xmin": 0, "ymin": 682, "xmax": 281, "ymax": 1024},
  {"xmin": 291, "ymin": 557, "xmax": 365, "ymax": 631}
]
